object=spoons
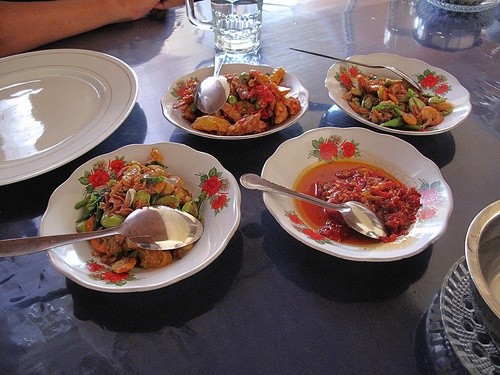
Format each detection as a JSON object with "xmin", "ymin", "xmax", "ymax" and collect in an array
[
  {"xmin": 289, "ymin": 47, "xmax": 422, "ymax": 92},
  {"xmin": 0, "ymin": 204, "xmax": 204, "ymax": 257},
  {"xmin": 239, "ymin": 173, "xmax": 385, "ymax": 239},
  {"xmin": 195, "ymin": 54, "xmax": 232, "ymax": 114}
]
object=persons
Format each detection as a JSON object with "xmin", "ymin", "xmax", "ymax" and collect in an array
[{"xmin": 0, "ymin": 0, "xmax": 185, "ymax": 57}]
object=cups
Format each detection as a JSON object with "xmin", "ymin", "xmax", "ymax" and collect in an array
[{"xmin": 185, "ymin": 0, "xmax": 264, "ymax": 56}]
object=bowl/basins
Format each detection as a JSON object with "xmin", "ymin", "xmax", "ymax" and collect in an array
[{"xmin": 465, "ymin": 198, "xmax": 500, "ymax": 321}]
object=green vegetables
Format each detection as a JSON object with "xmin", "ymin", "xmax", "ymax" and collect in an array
[
  {"xmin": 74, "ymin": 160, "xmax": 205, "ymax": 232},
  {"xmin": 372, "ymin": 88, "xmax": 449, "ymax": 130}
]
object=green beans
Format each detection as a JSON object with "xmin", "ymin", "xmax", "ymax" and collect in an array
[{"xmin": 228, "ymin": 71, "xmax": 271, "ymax": 109}]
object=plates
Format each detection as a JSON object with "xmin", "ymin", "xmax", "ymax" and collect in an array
[
  {"xmin": 426, "ymin": 0, "xmax": 500, "ymax": 13},
  {"xmin": 325, "ymin": 51, "xmax": 473, "ymax": 135},
  {"xmin": 0, "ymin": 49, "xmax": 139, "ymax": 188},
  {"xmin": 40, "ymin": 140, "xmax": 241, "ymax": 293},
  {"xmin": 261, "ymin": 126, "xmax": 453, "ymax": 264},
  {"xmin": 440, "ymin": 253, "xmax": 500, "ymax": 375},
  {"xmin": 158, "ymin": 63, "xmax": 310, "ymax": 140}
]
